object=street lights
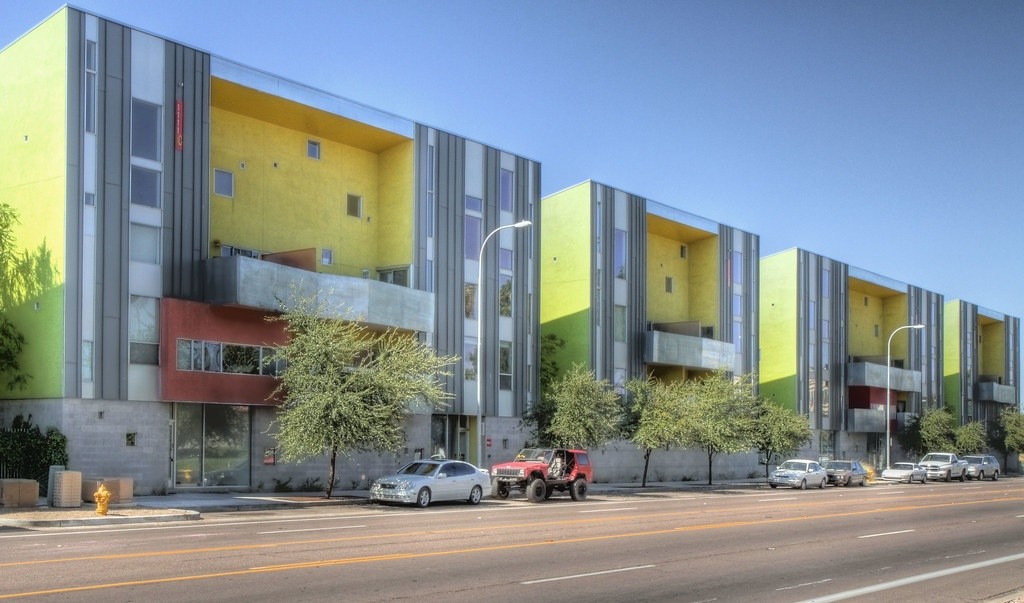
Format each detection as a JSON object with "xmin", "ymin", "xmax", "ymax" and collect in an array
[
  {"xmin": 476, "ymin": 220, "xmax": 531, "ymax": 470},
  {"xmin": 884, "ymin": 325, "xmax": 926, "ymax": 471}
]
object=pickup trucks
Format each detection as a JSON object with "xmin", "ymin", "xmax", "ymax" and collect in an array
[{"xmin": 919, "ymin": 452, "xmax": 969, "ymax": 483}]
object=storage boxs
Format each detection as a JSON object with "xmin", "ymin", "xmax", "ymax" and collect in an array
[
  {"xmin": 104, "ymin": 478, "xmax": 134, "ymax": 503},
  {"xmin": 47, "ymin": 465, "xmax": 65, "ymax": 506},
  {"xmin": 52, "ymin": 471, "xmax": 82, "ymax": 508},
  {"xmin": 81, "ymin": 478, "xmax": 107, "ymax": 503},
  {"xmin": 0, "ymin": 478, "xmax": 40, "ymax": 507}
]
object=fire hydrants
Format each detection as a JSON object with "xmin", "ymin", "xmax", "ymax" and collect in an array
[{"xmin": 93, "ymin": 484, "xmax": 112, "ymax": 516}]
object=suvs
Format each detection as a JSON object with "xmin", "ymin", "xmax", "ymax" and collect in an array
[{"xmin": 490, "ymin": 447, "xmax": 594, "ymax": 504}]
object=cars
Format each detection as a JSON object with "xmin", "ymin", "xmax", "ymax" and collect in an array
[
  {"xmin": 881, "ymin": 462, "xmax": 928, "ymax": 484},
  {"xmin": 371, "ymin": 458, "xmax": 493, "ymax": 507},
  {"xmin": 960, "ymin": 455, "xmax": 1000, "ymax": 481},
  {"xmin": 822, "ymin": 460, "xmax": 868, "ymax": 487},
  {"xmin": 767, "ymin": 459, "xmax": 829, "ymax": 490}
]
